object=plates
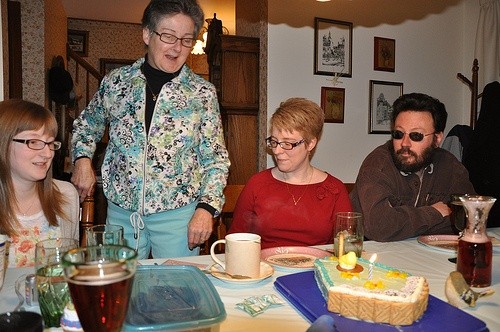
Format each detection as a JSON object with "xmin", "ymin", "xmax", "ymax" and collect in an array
[
  {"xmin": 418, "ymin": 234, "xmax": 460, "ymax": 253},
  {"xmin": 210, "ymin": 261, "xmax": 274, "ymax": 285},
  {"xmin": 261, "ymin": 246, "xmax": 334, "ymax": 269},
  {"xmin": 459, "ymin": 231, "xmax": 500, "ymax": 246}
]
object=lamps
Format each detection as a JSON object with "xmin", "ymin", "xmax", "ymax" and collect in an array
[{"xmin": 191, "ymin": 19, "xmax": 229, "ymax": 55}]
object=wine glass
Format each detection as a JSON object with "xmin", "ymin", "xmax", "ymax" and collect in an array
[{"xmin": 447, "ymin": 194, "xmax": 467, "ymax": 263}]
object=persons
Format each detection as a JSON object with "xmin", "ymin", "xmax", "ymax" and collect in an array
[
  {"xmin": 70, "ymin": 0, "xmax": 231, "ymax": 260},
  {"xmin": 0, "ymin": 99, "xmax": 79, "ymax": 267},
  {"xmin": 228, "ymin": 98, "xmax": 353, "ymax": 250},
  {"xmin": 350, "ymin": 93, "xmax": 476, "ymax": 241}
]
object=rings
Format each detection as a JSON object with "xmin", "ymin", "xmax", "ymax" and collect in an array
[{"xmin": 200, "ymin": 239, "xmax": 204, "ymax": 243}]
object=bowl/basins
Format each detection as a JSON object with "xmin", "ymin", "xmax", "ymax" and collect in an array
[{"xmin": 118, "ymin": 265, "xmax": 227, "ymax": 332}]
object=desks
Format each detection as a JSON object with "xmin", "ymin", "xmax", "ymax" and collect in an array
[{"xmin": 0, "ymin": 227, "xmax": 500, "ymax": 332}]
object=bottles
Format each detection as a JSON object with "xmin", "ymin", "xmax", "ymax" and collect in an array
[
  {"xmin": 457, "ymin": 195, "xmax": 496, "ymax": 289},
  {"xmin": 25, "ymin": 274, "xmax": 38, "ymax": 306}
]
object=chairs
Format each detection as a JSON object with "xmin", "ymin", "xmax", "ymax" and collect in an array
[
  {"xmin": 82, "ymin": 187, "xmax": 108, "ymax": 247},
  {"xmin": 203, "ymin": 184, "xmax": 246, "ymax": 255}
]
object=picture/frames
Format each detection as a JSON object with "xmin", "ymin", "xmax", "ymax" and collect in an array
[
  {"xmin": 368, "ymin": 80, "xmax": 403, "ymax": 134},
  {"xmin": 321, "ymin": 86, "xmax": 345, "ymax": 123},
  {"xmin": 100, "ymin": 58, "xmax": 137, "ymax": 76},
  {"xmin": 374, "ymin": 36, "xmax": 396, "ymax": 72},
  {"xmin": 314, "ymin": 17, "xmax": 352, "ymax": 78},
  {"xmin": 67, "ymin": 29, "xmax": 89, "ymax": 57}
]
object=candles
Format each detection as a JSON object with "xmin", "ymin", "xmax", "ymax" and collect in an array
[
  {"xmin": 368, "ymin": 253, "xmax": 377, "ymax": 281},
  {"xmin": 339, "ymin": 232, "xmax": 344, "ymax": 259}
]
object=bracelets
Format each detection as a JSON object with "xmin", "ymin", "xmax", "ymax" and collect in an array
[{"xmin": 195, "ymin": 203, "xmax": 218, "ymax": 215}]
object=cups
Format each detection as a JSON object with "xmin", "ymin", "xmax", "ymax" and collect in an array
[
  {"xmin": 0, "ymin": 312, "xmax": 44, "ymax": 332},
  {"xmin": 86, "ymin": 224, "xmax": 124, "ymax": 248},
  {"xmin": 334, "ymin": 212, "xmax": 364, "ymax": 260},
  {"xmin": 211, "ymin": 233, "xmax": 261, "ymax": 278},
  {"xmin": 63, "ymin": 245, "xmax": 138, "ymax": 332},
  {"xmin": 35, "ymin": 238, "xmax": 80, "ymax": 332},
  {"xmin": 0, "ymin": 234, "xmax": 9, "ymax": 291}
]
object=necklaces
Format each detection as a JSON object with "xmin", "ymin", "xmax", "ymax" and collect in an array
[
  {"xmin": 284, "ymin": 165, "xmax": 313, "ymax": 205},
  {"xmin": 147, "ymin": 82, "xmax": 158, "ymax": 101},
  {"xmin": 23, "ymin": 198, "xmax": 35, "ymax": 216}
]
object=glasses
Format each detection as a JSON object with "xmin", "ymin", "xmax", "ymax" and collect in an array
[
  {"xmin": 12, "ymin": 139, "xmax": 61, "ymax": 151},
  {"xmin": 265, "ymin": 137, "xmax": 306, "ymax": 150},
  {"xmin": 391, "ymin": 130, "xmax": 436, "ymax": 142},
  {"xmin": 152, "ymin": 30, "xmax": 196, "ymax": 48}
]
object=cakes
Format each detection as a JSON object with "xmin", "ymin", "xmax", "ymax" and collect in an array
[{"xmin": 315, "ymin": 251, "xmax": 430, "ymax": 326}]
